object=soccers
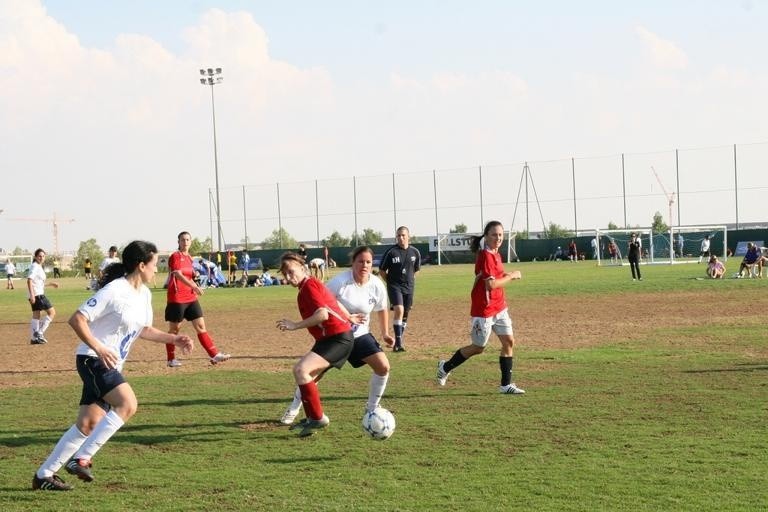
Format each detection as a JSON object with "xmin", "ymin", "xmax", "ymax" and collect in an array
[{"xmin": 363, "ymin": 408, "xmax": 395, "ymax": 443}]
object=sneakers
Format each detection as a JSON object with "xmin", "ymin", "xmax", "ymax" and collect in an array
[
  {"xmin": 166, "ymin": 360, "xmax": 184, "ymax": 367},
  {"xmin": 209, "ymin": 352, "xmax": 230, "ymax": 364},
  {"xmin": 280, "ymin": 405, "xmax": 297, "ymax": 423},
  {"xmin": 298, "ymin": 415, "xmax": 331, "ymax": 438},
  {"xmin": 33, "ymin": 328, "xmax": 47, "ymax": 342},
  {"xmin": 288, "ymin": 417, "xmax": 312, "ymax": 431},
  {"xmin": 436, "ymin": 360, "xmax": 450, "ymax": 389},
  {"xmin": 29, "ymin": 338, "xmax": 43, "ymax": 344},
  {"xmin": 64, "ymin": 457, "xmax": 95, "ymax": 483},
  {"xmin": 500, "ymin": 383, "xmax": 527, "ymax": 395},
  {"xmin": 31, "ymin": 472, "xmax": 72, "ymax": 491},
  {"xmin": 393, "ymin": 345, "xmax": 407, "ymax": 351}
]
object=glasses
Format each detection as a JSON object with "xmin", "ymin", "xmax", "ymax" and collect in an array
[{"xmin": 630, "ymin": 234, "xmax": 637, "ymax": 238}]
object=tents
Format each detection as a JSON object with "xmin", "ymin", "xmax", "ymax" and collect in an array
[{"xmin": 163, "ymin": 256, "xmax": 225, "ymax": 288}]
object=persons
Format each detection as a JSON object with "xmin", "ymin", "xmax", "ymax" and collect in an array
[
  {"xmin": 274, "ymin": 251, "xmax": 354, "ymax": 438},
  {"xmin": 297, "ymin": 244, "xmax": 307, "ymax": 268},
  {"xmin": 239, "ymin": 249, "xmax": 249, "ymax": 277},
  {"xmin": 568, "ymin": 239, "xmax": 577, "ymax": 263},
  {"xmin": 84, "ymin": 257, "xmax": 92, "ymax": 282},
  {"xmin": 697, "ymin": 235, "xmax": 710, "ymax": 264},
  {"xmin": 214, "ymin": 250, "xmax": 222, "ymax": 266},
  {"xmin": 705, "ymin": 255, "xmax": 726, "ymax": 279},
  {"xmin": 197, "ymin": 259, "xmax": 221, "ymax": 289},
  {"xmin": 3, "ymin": 258, "xmax": 15, "ymax": 289},
  {"xmin": 591, "ymin": 237, "xmax": 599, "ymax": 259},
  {"xmin": 434, "ymin": 220, "xmax": 526, "ymax": 395},
  {"xmin": 548, "ymin": 245, "xmax": 563, "ymax": 262},
  {"xmin": 32, "ymin": 241, "xmax": 193, "ymax": 490},
  {"xmin": 308, "ymin": 258, "xmax": 325, "ymax": 283},
  {"xmin": 746, "ymin": 245, "xmax": 768, "ymax": 280},
  {"xmin": 97, "ymin": 246, "xmax": 121, "ymax": 275},
  {"xmin": 280, "ymin": 246, "xmax": 396, "ymax": 427},
  {"xmin": 377, "ymin": 224, "xmax": 421, "ymax": 353},
  {"xmin": 627, "ymin": 232, "xmax": 643, "ymax": 282},
  {"xmin": 636, "ymin": 234, "xmax": 643, "ymax": 261},
  {"xmin": 259, "ymin": 267, "xmax": 271, "ymax": 282},
  {"xmin": 735, "ymin": 243, "xmax": 755, "ymax": 278},
  {"xmin": 52, "ymin": 255, "xmax": 61, "ymax": 277},
  {"xmin": 25, "ymin": 247, "xmax": 55, "ymax": 346},
  {"xmin": 677, "ymin": 233, "xmax": 684, "ymax": 257},
  {"xmin": 607, "ymin": 239, "xmax": 616, "ymax": 264},
  {"xmin": 227, "ymin": 251, "xmax": 236, "ymax": 288}
]
